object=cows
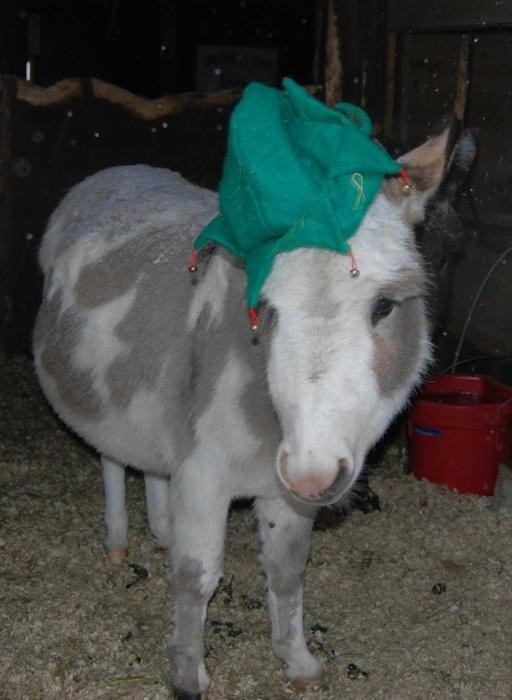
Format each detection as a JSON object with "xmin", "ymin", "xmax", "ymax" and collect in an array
[{"xmin": 30, "ymin": 125, "xmax": 453, "ymax": 700}]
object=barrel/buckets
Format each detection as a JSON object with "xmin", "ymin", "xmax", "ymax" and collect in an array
[{"xmin": 402, "ymin": 372, "xmax": 512, "ymax": 499}]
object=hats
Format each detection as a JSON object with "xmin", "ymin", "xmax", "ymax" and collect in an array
[{"xmin": 186, "ymin": 75, "xmax": 411, "ymax": 336}]
